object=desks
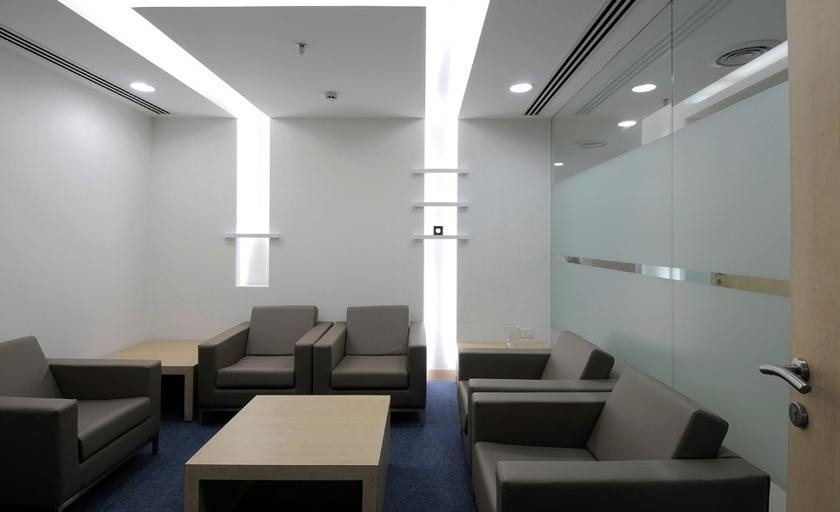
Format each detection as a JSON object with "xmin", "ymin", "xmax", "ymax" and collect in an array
[
  {"xmin": 106, "ymin": 338, "xmax": 206, "ymax": 422},
  {"xmin": 184, "ymin": 394, "xmax": 392, "ymax": 511}
]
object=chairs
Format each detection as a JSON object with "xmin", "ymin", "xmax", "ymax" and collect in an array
[
  {"xmin": 0, "ymin": 335, "xmax": 162, "ymax": 512},
  {"xmin": 457, "ymin": 329, "xmax": 619, "ymax": 465},
  {"xmin": 197, "ymin": 304, "xmax": 334, "ymax": 426},
  {"xmin": 469, "ymin": 364, "xmax": 771, "ymax": 511},
  {"xmin": 312, "ymin": 305, "xmax": 428, "ymax": 411}
]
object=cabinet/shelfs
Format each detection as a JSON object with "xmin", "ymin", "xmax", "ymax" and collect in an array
[{"xmin": 411, "ymin": 166, "xmax": 469, "ymax": 242}]
